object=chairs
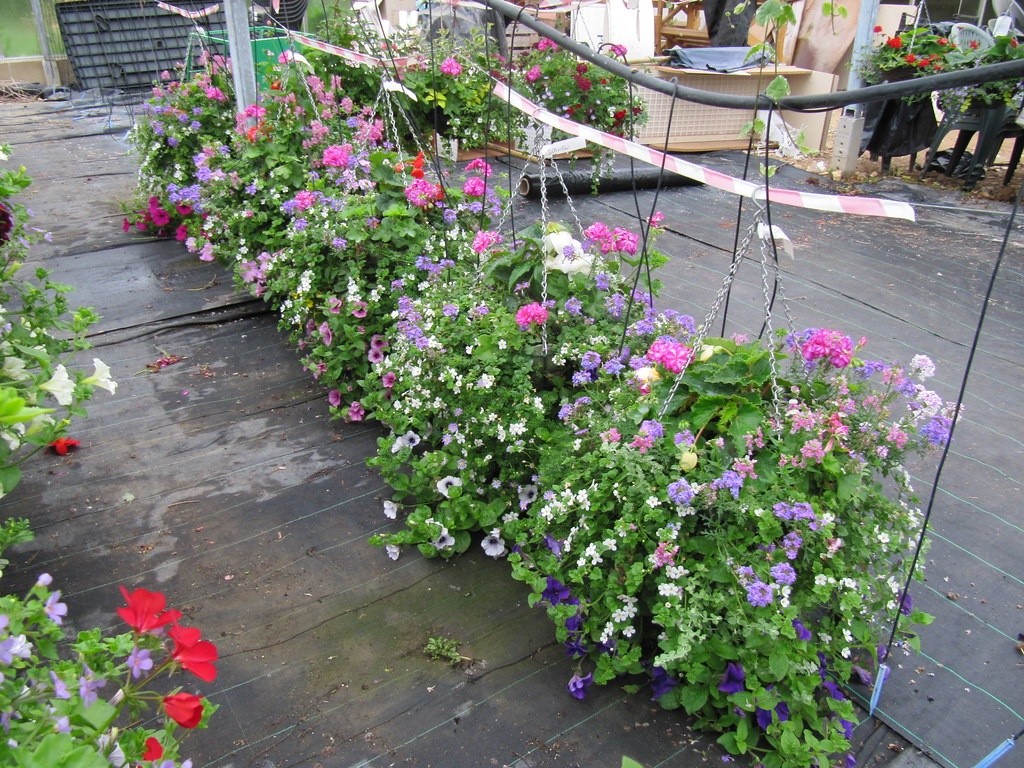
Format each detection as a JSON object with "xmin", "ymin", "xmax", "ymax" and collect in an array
[{"xmin": 921, "ymin": 23, "xmax": 1024, "ymax": 190}]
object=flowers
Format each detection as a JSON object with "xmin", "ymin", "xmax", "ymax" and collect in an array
[{"xmin": 1, "ymin": 21, "xmax": 1023, "ymax": 768}]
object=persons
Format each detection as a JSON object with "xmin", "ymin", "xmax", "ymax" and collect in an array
[{"xmin": 703, "ymin": 0, "xmax": 758, "ymax": 48}]
